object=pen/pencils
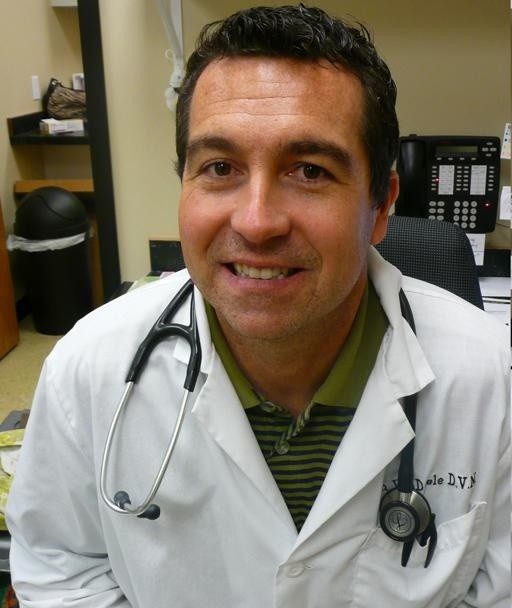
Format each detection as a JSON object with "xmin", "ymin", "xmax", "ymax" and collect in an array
[{"xmin": 401, "ymin": 513, "xmax": 437, "ymax": 568}]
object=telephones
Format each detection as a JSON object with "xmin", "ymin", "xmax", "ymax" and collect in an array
[{"xmin": 396, "ymin": 134, "xmax": 501, "ymax": 233}]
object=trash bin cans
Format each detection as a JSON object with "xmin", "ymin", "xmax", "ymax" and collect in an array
[{"xmin": 7, "ymin": 186, "xmax": 93, "ymax": 335}]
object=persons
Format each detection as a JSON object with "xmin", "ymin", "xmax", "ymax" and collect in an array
[{"xmin": 4, "ymin": 2, "xmax": 512, "ymax": 608}]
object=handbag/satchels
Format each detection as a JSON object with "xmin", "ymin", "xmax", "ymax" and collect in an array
[{"xmin": 43, "ymin": 78, "xmax": 87, "ymax": 119}]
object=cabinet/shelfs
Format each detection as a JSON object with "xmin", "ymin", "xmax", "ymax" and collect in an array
[{"xmin": 10, "ymin": 180, "xmax": 104, "ymax": 309}]
